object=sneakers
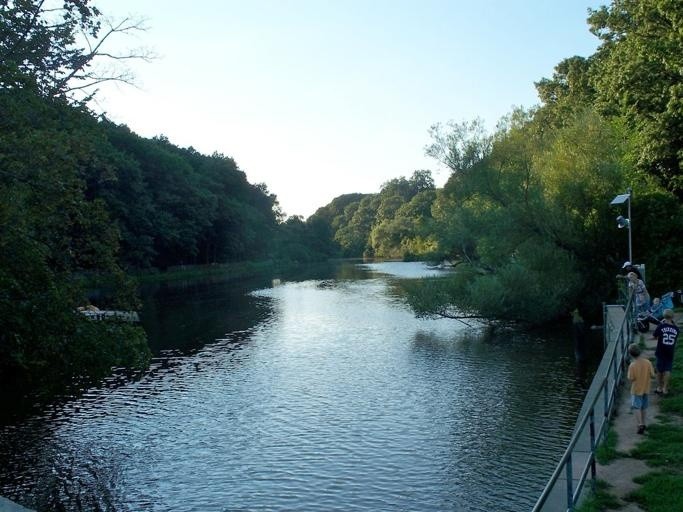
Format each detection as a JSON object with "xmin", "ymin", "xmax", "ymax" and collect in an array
[{"xmin": 637, "ymin": 424, "xmax": 646, "ymax": 435}]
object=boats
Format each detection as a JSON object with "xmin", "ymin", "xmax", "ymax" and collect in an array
[{"xmin": 73, "ymin": 303, "xmax": 139, "ymax": 325}]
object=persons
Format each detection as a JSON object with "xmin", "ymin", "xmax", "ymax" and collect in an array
[
  {"xmin": 624, "ymin": 342, "xmax": 657, "ymax": 435},
  {"xmin": 627, "ymin": 271, "xmax": 650, "ymax": 314},
  {"xmin": 622, "ymin": 261, "xmax": 644, "ymax": 286},
  {"xmin": 651, "ymin": 309, "xmax": 680, "ymax": 396},
  {"xmin": 651, "ymin": 298, "xmax": 660, "ymax": 314}
]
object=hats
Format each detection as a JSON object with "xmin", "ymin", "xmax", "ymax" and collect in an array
[{"xmin": 621, "ymin": 261, "xmax": 632, "ymax": 268}]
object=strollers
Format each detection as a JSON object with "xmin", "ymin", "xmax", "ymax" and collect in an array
[{"xmin": 637, "ymin": 291, "xmax": 674, "ymax": 333}]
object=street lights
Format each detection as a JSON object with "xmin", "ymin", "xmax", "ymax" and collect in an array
[{"xmin": 611, "ymin": 187, "xmax": 633, "ymax": 266}]
